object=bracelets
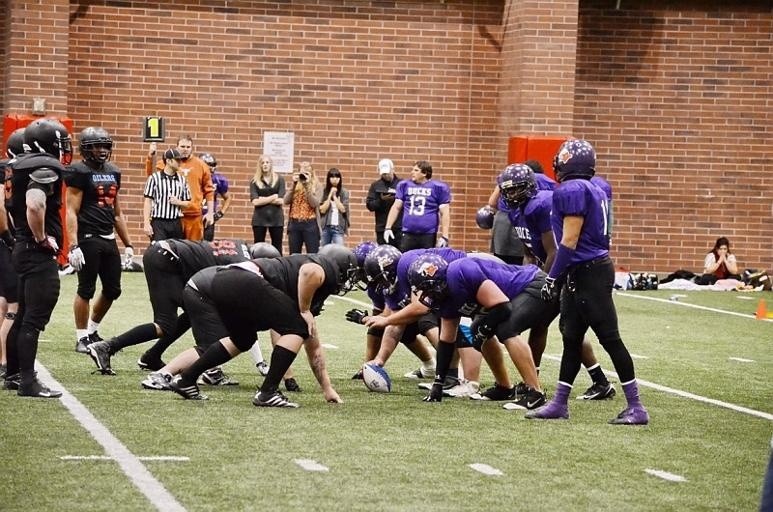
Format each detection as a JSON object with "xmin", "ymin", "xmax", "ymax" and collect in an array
[
  {"xmin": 32, "ymin": 232, "xmax": 47, "ymax": 243},
  {"xmin": 125, "ymin": 245, "xmax": 134, "ymax": 249}
]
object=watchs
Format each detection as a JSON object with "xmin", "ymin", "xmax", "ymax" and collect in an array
[{"xmin": 68, "ymin": 245, "xmax": 79, "ymax": 251}]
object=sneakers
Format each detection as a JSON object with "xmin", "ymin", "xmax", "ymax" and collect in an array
[
  {"xmin": 442, "ymin": 381, "xmax": 480, "ymax": 398},
  {"xmin": 252, "ymin": 390, "xmax": 301, "ymax": 409},
  {"xmin": 502, "ymin": 386, "xmax": 548, "ymax": 410},
  {"xmin": 403, "ymin": 366, "xmax": 464, "ymax": 391},
  {"xmin": 0, "ymin": 364, "xmax": 62, "ymax": 397},
  {"xmin": 468, "ymin": 382, "xmax": 515, "ymax": 401},
  {"xmin": 75, "ymin": 330, "xmax": 116, "ymax": 375},
  {"xmin": 137, "ymin": 351, "xmax": 166, "ymax": 371},
  {"xmin": 516, "ymin": 381, "xmax": 530, "ymax": 397},
  {"xmin": 575, "ymin": 382, "xmax": 616, "ymax": 401},
  {"xmin": 523, "ymin": 400, "xmax": 569, "ymax": 419},
  {"xmin": 140, "ymin": 366, "xmax": 239, "ymax": 401},
  {"xmin": 606, "ymin": 407, "xmax": 648, "ymax": 424}
]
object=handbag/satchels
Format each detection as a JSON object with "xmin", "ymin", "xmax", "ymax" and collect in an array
[{"xmin": 626, "ymin": 271, "xmax": 658, "ymax": 290}]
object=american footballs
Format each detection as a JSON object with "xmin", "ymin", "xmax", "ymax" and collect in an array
[{"xmin": 361, "ymin": 363, "xmax": 390, "ymax": 392}]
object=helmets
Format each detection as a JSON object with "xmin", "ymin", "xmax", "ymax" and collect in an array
[
  {"xmin": 363, "ymin": 245, "xmax": 403, "ymax": 296},
  {"xmin": 199, "ymin": 152, "xmax": 217, "ymax": 174},
  {"xmin": 351, "ymin": 240, "xmax": 379, "ymax": 291},
  {"xmin": 407, "ymin": 252, "xmax": 448, "ymax": 309},
  {"xmin": 6, "ymin": 119, "xmax": 73, "ymax": 165},
  {"xmin": 552, "ymin": 138, "xmax": 596, "ymax": 183},
  {"xmin": 78, "ymin": 126, "xmax": 112, "ymax": 164},
  {"xmin": 250, "ymin": 241, "xmax": 281, "ymax": 259},
  {"xmin": 498, "ymin": 163, "xmax": 537, "ymax": 210},
  {"xmin": 317, "ymin": 243, "xmax": 359, "ymax": 296}
]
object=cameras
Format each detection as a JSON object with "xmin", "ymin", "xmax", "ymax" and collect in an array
[{"xmin": 298, "ymin": 172, "xmax": 308, "ymax": 183}]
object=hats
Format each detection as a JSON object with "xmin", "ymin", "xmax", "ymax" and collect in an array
[
  {"xmin": 162, "ymin": 148, "xmax": 184, "ymax": 159},
  {"xmin": 378, "ymin": 159, "xmax": 393, "ymax": 175}
]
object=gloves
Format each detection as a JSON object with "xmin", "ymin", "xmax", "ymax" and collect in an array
[
  {"xmin": 345, "ymin": 309, "xmax": 367, "ymax": 324},
  {"xmin": 68, "ymin": 246, "xmax": 85, "ymax": 272},
  {"xmin": 475, "ymin": 205, "xmax": 496, "ymax": 229},
  {"xmin": 2, "ymin": 238, "xmax": 16, "ymax": 251},
  {"xmin": 435, "ymin": 235, "xmax": 448, "ymax": 248},
  {"xmin": 34, "ymin": 232, "xmax": 59, "ymax": 253},
  {"xmin": 124, "ymin": 246, "xmax": 133, "ymax": 266},
  {"xmin": 213, "ymin": 210, "xmax": 223, "ymax": 221},
  {"xmin": 422, "ymin": 382, "xmax": 442, "ymax": 402},
  {"xmin": 540, "ymin": 275, "xmax": 556, "ymax": 301},
  {"xmin": 471, "ymin": 329, "xmax": 487, "ymax": 353},
  {"xmin": 383, "ymin": 228, "xmax": 394, "ymax": 243}
]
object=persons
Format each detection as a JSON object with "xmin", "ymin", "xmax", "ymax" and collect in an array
[
  {"xmin": 345, "ymin": 240, "xmax": 561, "ymax": 410},
  {"xmin": 85, "ymin": 238, "xmax": 362, "ymax": 408},
  {"xmin": 702, "ymin": 237, "xmax": 752, "ymax": 290},
  {"xmin": 319, "ymin": 168, "xmax": 350, "ymax": 248},
  {"xmin": 366, "ymin": 158, "xmax": 403, "ymax": 244},
  {"xmin": 249, "ymin": 153, "xmax": 286, "ymax": 256},
  {"xmin": 476, "ymin": 159, "xmax": 617, "ymax": 400},
  {"xmin": 283, "ymin": 161, "xmax": 324, "ymax": 255},
  {"xmin": 384, "ymin": 162, "xmax": 452, "ymax": 254},
  {"xmin": 143, "ymin": 135, "xmax": 232, "ymax": 242},
  {"xmin": 63, "ymin": 127, "xmax": 133, "ymax": 354},
  {"xmin": 524, "ymin": 138, "xmax": 648, "ymax": 423},
  {"xmin": 2, "ymin": 119, "xmax": 73, "ymax": 398}
]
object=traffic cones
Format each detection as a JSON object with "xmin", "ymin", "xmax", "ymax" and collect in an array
[{"xmin": 752, "ymin": 294, "xmax": 768, "ymax": 322}]
